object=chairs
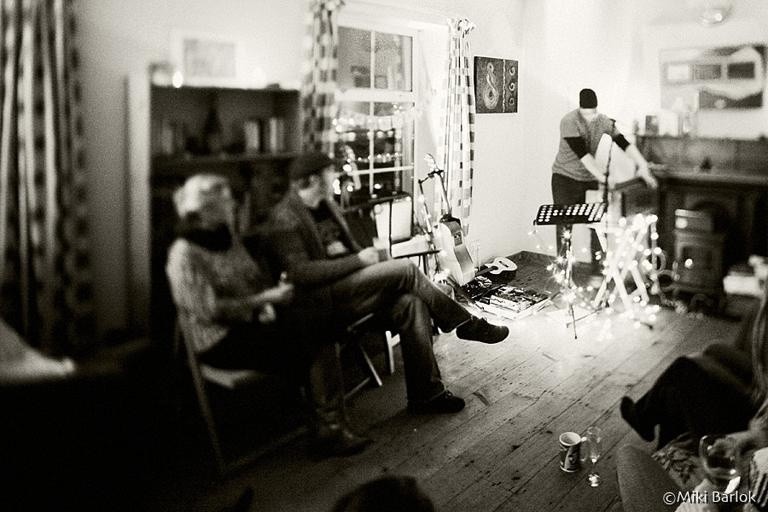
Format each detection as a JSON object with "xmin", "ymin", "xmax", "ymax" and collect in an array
[{"xmin": 164, "ymin": 262, "xmax": 312, "ymax": 477}]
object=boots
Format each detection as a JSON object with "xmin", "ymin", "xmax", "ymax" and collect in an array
[{"xmin": 307, "ymin": 358, "xmax": 378, "ymax": 461}]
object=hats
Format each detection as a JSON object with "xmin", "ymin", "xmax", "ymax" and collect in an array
[
  {"xmin": 289, "ymin": 150, "xmax": 343, "ymax": 181},
  {"xmin": 579, "ymin": 89, "xmax": 598, "ymax": 109}
]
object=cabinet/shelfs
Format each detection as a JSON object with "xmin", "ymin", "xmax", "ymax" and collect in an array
[{"xmin": 127, "ymin": 69, "xmax": 305, "ymax": 338}]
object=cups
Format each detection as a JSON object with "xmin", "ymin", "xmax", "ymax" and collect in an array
[
  {"xmin": 587, "ymin": 427, "xmax": 603, "ymax": 459},
  {"xmin": 559, "ymin": 432, "xmax": 590, "ymax": 472}
]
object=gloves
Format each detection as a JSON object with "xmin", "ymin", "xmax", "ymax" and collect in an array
[
  {"xmin": 625, "ymin": 144, "xmax": 658, "ymax": 191},
  {"xmin": 581, "ymin": 153, "xmax": 616, "ymax": 189}
]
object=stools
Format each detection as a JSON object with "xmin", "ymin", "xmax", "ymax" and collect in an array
[{"xmin": 330, "ymin": 312, "xmax": 384, "ymax": 424}]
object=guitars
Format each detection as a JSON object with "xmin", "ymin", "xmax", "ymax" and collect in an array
[{"xmin": 476, "ymin": 255, "xmax": 519, "ymax": 280}]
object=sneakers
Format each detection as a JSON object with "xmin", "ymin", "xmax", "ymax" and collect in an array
[
  {"xmin": 406, "ymin": 390, "xmax": 465, "ymax": 416},
  {"xmin": 456, "ymin": 316, "xmax": 509, "ymax": 343}
]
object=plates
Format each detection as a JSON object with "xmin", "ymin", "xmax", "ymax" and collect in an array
[{"xmin": 700, "ymin": 434, "xmax": 740, "ymax": 479}]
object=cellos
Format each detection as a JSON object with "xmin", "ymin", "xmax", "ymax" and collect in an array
[{"xmin": 424, "ymin": 152, "xmax": 475, "ymax": 287}]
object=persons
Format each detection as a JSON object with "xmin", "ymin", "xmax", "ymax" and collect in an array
[
  {"xmin": 619, "ymin": 338, "xmax": 755, "ymax": 442},
  {"xmin": 166, "ymin": 173, "xmax": 370, "ymax": 456},
  {"xmin": 0, "ymin": 317, "xmax": 165, "ymax": 450},
  {"xmin": 551, "ymin": 89, "xmax": 658, "ymax": 275},
  {"xmin": 270, "ymin": 151, "xmax": 509, "ymax": 414}
]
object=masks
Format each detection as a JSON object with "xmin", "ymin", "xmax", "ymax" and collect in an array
[{"xmin": 580, "ymin": 109, "xmax": 597, "ymax": 122}]
